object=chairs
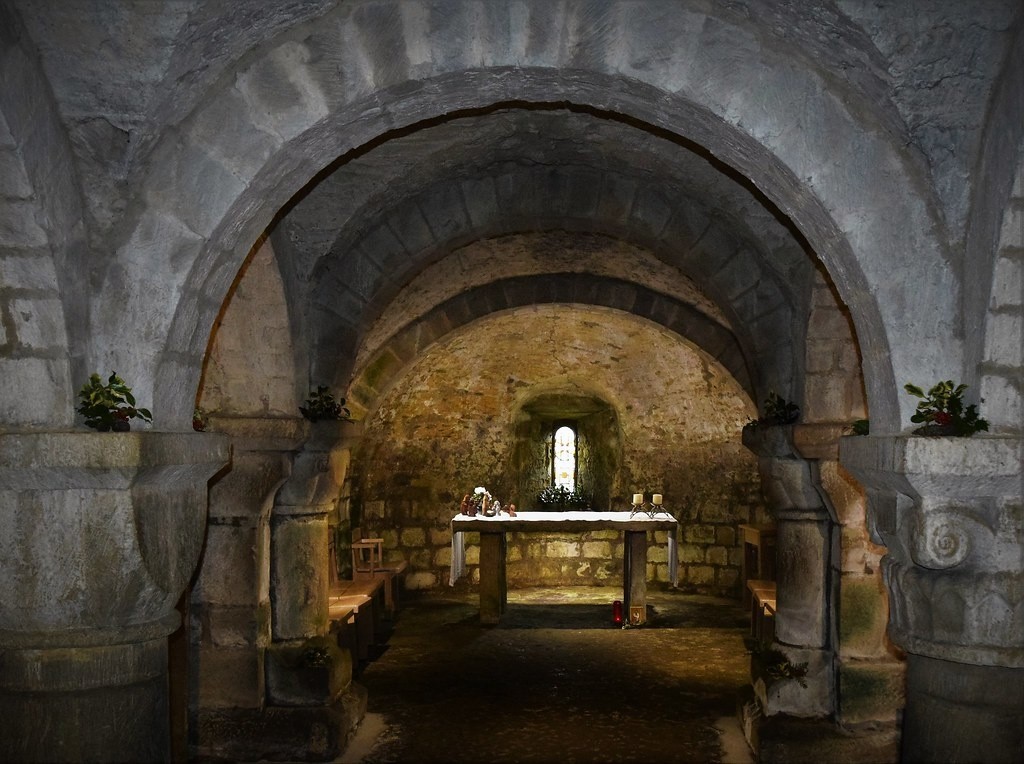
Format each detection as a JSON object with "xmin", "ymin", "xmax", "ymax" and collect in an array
[
  {"xmin": 746, "ymin": 579, "xmax": 776, "ymax": 640},
  {"xmin": 329, "ymin": 595, "xmax": 374, "ymax": 660},
  {"xmin": 329, "ymin": 607, "xmax": 360, "ymax": 669},
  {"xmin": 327, "ymin": 528, "xmax": 383, "ymax": 634},
  {"xmin": 351, "ymin": 527, "xmax": 407, "ymax": 614}
]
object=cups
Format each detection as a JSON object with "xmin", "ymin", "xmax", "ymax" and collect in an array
[{"xmin": 613, "ymin": 601, "xmax": 622, "ymax": 627}]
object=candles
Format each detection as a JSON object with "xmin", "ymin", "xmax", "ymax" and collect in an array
[
  {"xmin": 633, "ymin": 493, "xmax": 643, "ymax": 504},
  {"xmin": 653, "ymin": 494, "xmax": 662, "ymax": 504}
]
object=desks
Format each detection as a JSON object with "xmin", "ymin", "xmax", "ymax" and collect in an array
[
  {"xmin": 738, "ymin": 523, "xmax": 776, "ymax": 612},
  {"xmin": 452, "ymin": 510, "xmax": 677, "ymax": 627}
]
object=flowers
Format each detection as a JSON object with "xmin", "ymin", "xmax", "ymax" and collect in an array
[
  {"xmin": 193, "ymin": 409, "xmax": 207, "ymax": 432},
  {"xmin": 470, "ymin": 487, "xmax": 492, "ymax": 506},
  {"xmin": 74, "ymin": 370, "xmax": 155, "ymax": 432},
  {"xmin": 904, "ymin": 380, "xmax": 991, "ymax": 438},
  {"xmin": 745, "ymin": 392, "xmax": 800, "ymax": 432}
]
